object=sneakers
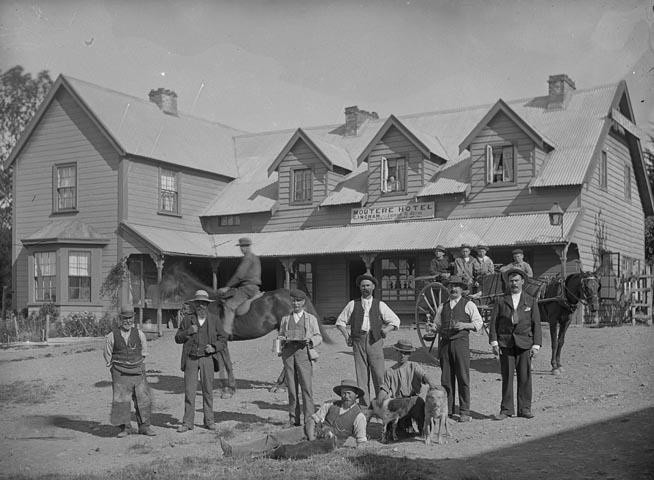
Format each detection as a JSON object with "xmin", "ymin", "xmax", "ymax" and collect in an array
[
  {"xmin": 144, "ymin": 430, "xmax": 157, "ymax": 436},
  {"xmin": 177, "ymin": 425, "xmax": 188, "ymax": 432},
  {"xmin": 495, "ymin": 413, "xmax": 507, "ymax": 420},
  {"xmin": 208, "ymin": 423, "xmax": 215, "ymax": 429},
  {"xmin": 519, "ymin": 411, "xmax": 534, "ymax": 417},
  {"xmin": 220, "ymin": 437, "xmax": 232, "ymax": 456},
  {"xmin": 118, "ymin": 431, "xmax": 128, "ymax": 437},
  {"xmin": 459, "ymin": 415, "xmax": 468, "ymax": 422}
]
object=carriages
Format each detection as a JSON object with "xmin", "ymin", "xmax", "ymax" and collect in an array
[{"xmin": 414, "ymin": 267, "xmax": 605, "ymax": 377}]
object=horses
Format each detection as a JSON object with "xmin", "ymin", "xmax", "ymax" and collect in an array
[
  {"xmin": 156, "ymin": 261, "xmax": 334, "ymax": 399},
  {"xmin": 521, "ymin": 264, "xmax": 604, "ymax": 375}
]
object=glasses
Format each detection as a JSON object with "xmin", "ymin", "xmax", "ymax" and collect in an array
[{"xmin": 120, "ymin": 305, "xmax": 136, "ymax": 317}]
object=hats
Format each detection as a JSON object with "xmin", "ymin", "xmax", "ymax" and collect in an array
[
  {"xmin": 236, "ymin": 237, "xmax": 254, "ymax": 245},
  {"xmin": 333, "ymin": 379, "xmax": 365, "ymax": 398},
  {"xmin": 443, "ymin": 275, "xmax": 468, "ymax": 290},
  {"xmin": 188, "ymin": 290, "xmax": 216, "ymax": 302},
  {"xmin": 356, "ymin": 273, "xmax": 378, "ymax": 286},
  {"xmin": 393, "ymin": 338, "xmax": 417, "ymax": 353},
  {"xmin": 512, "ymin": 249, "xmax": 524, "ymax": 254},
  {"xmin": 290, "ymin": 289, "xmax": 306, "ymax": 300},
  {"xmin": 433, "ymin": 243, "xmax": 489, "ymax": 251},
  {"xmin": 505, "ymin": 267, "xmax": 528, "ymax": 283}
]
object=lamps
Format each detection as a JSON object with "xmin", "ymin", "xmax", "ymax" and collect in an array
[{"xmin": 549, "ymin": 200, "xmax": 566, "ymax": 239}]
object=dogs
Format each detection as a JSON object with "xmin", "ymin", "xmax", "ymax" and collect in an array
[
  {"xmin": 424, "ymin": 385, "xmax": 452, "ymax": 446},
  {"xmin": 251, "ymin": 436, "xmax": 335, "ymax": 460},
  {"xmin": 364, "ymin": 396, "xmax": 424, "ymax": 444}
]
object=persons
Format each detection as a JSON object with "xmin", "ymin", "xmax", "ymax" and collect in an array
[
  {"xmin": 332, "ymin": 272, "xmax": 401, "ymax": 405},
  {"xmin": 276, "ymin": 289, "xmax": 323, "ymax": 431},
  {"xmin": 488, "ymin": 265, "xmax": 544, "ymax": 420},
  {"xmin": 427, "ymin": 274, "xmax": 485, "ymax": 423},
  {"xmin": 215, "ymin": 236, "xmax": 263, "ymax": 335},
  {"xmin": 451, "ymin": 242, "xmax": 479, "ymax": 294},
  {"xmin": 387, "ymin": 175, "xmax": 401, "ymax": 192},
  {"xmin": 474, "ymin": 244, "xmax": 495, "ymax": 277},
  {"xmin": 174, "ymin": 287, "xmax": 227, "ymax": 434},
  {"xmin": 497, "ymin": 248, "xmax": 535, "ymax": 279},
  {"xmin": 425, "ymin": 242, "xmax": 447, "ymax": 271},
  {"xmin": 220, "ymin": 378, "xmax": 367, "ymax": 460},
  {"xmin": 376, "ymin": 336, "xmax": 443, "ymax": 441},
  {"xmin": 102, "ymin": 306, "xmax": 156, "ymax": 437}
]
